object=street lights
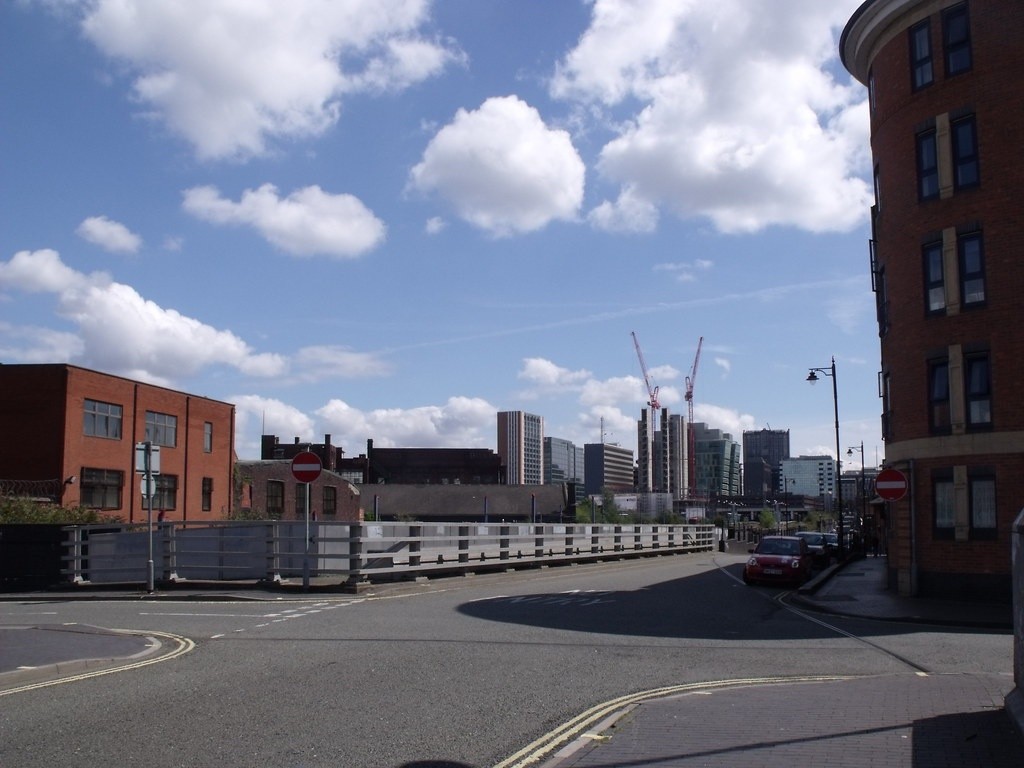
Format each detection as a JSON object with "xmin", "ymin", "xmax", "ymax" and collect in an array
[
  {"xmin": 806, "ymin": 355, "xmax": 844, "ymax": 555},
  {"xmin": 847, "ymin": 440, "xmax": 865, "ymax": 542}
]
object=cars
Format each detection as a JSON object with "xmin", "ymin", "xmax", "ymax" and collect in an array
[
  {"xmin": 795, "ymin": 531, "xmax": 828, "ymax": 570},
  {"xmin": 836, "ymin": 516, "xmax": 856, "ymax": 546},
  {"xmin": 743, "ymin": 535, "xmax": 813, "ymax": 587},
  {"xmin": 821, "ymin": 532, "xmax": 839, "ymax": 560}
]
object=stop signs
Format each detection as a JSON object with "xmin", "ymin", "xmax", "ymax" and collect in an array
[
  {"xmin": 290, "ymin": 451, "xmax": 323, "ymax": 483},
  {"xmin": 875, "ymin": 467, "xmax": 909, "ymax": 502}
]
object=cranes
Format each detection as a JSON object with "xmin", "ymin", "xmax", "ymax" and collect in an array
[
  {"xmin": 632, "ymin": 331, "xmax": 661, "ymax": 495},
  {"xmin": 684, "ymin": 336, "xmax": 703, "ymax": 500}
]
